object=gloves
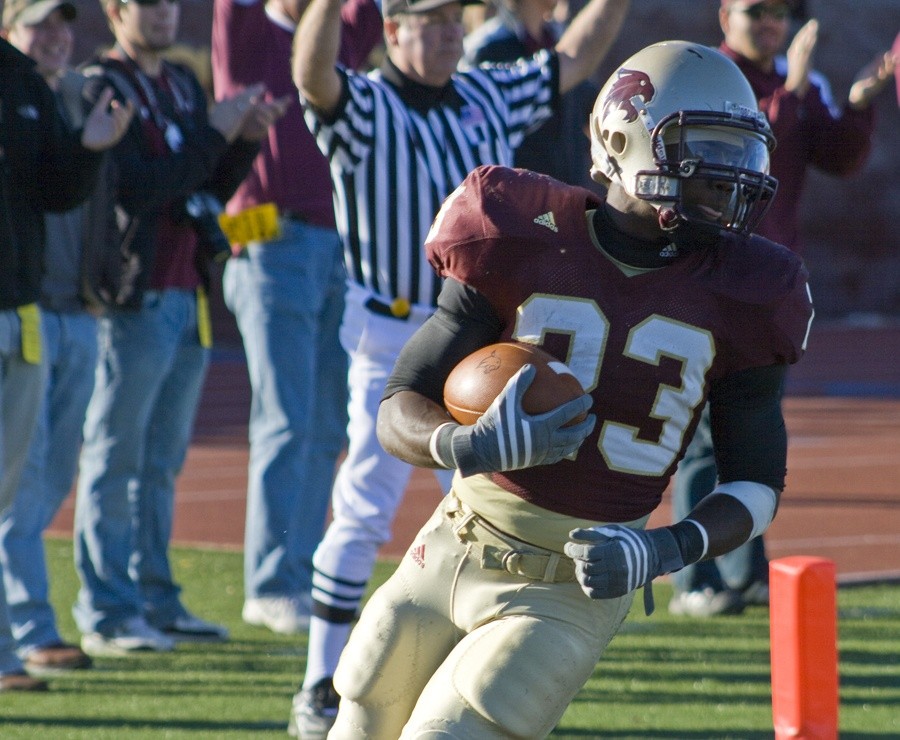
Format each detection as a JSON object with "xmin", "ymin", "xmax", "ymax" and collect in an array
[
  {"xmin": 564, "ymin": 524, "xmax": 684, "ymax": 600},
  {"xmin": 452, "ymin": 364, "xmax": 596, "ymax": 478}
]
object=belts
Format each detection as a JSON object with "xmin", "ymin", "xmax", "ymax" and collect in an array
[
  {"xmin": 365, "ymin": 296, "xmax": 411, "ymax": 321},
  {"xmin": 451, "ymin": 509, "xmax": 575, "ymax": 581}
]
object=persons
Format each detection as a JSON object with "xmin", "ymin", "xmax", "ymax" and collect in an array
[
  {"xmin": 1, "ymin": 2, "xmax": 631, "ymax": 694},
  {"xmin": 668, "ymin": 2, "xmax": 897, "ymax": 618},
  {"xmin": 325, "ymin": 40, "xmax": 816, "ymax": 739}
]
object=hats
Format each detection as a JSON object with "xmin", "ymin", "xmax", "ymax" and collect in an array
[
  {"xmin": 382, "ymin": 0, "xmax": 484, "ymax": 17},
  {"xmin": 2, "ymin": 0, "xmax": 76, "ymax": 28}
]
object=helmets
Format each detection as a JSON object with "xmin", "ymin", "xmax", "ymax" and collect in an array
[{"xmin": 589, "ymin": 40, "xmax": 778, "ymax": 238}]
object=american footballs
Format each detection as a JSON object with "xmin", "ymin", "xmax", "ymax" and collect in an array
[{"xmin": 443, "ymin": 342, "xmax": 588, "ymax": 431}]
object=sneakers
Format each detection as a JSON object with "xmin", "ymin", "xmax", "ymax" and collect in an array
[
  {"xmin": 242, "ymin": 589, "xmax": 311, "ymax": 632},
  {"xmin": 287, "ymin": 675, "xmax": 340, "ymax": 740},
  {"xmin": 81, "ymin": 618, "xmax": 175, "ymax": 654},
  {"xmin": 147, "ymin": 607, "xmax": 226, "ymax": 644}
]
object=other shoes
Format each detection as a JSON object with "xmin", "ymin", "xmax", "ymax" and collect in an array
[
  {"xmin": 29, "ymin": 641, "xmax": 93, "ymax": 668},
  {"xmin": 715, "ymin": 558, "xmax": 768, "ymax": 604},
  {"xmin": 668, "ymin": 585, "xmax": 744, "ymax": 617},
  {"xmin": 0, "ymin": 672, "xmax": 45, "ymax": 693}
]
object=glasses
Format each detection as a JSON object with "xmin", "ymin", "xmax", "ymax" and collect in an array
[{"xmin": 738, "ymin": 3, "xmax": 788, "ymax": 20}]
